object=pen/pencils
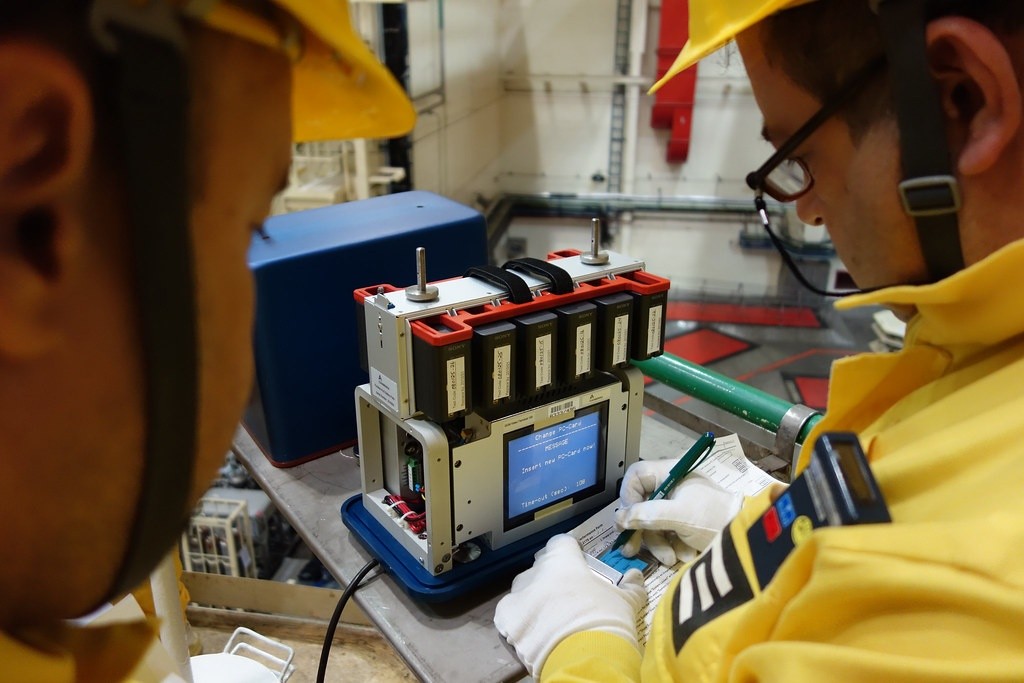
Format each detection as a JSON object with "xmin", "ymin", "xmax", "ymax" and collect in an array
[{"xmin": 608, "ymin": 431, "xmax": 717, "ymax": 556}]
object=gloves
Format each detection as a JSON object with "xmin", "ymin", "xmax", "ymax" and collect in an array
[
  {"xmin": 613, "ymin": 459, "xmax": 743, "ymax": 566},
  {"xmin": 493, "ymin": 533, "xmax": 647, "ymax": 683}
]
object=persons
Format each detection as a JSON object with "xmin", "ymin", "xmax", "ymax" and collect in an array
[
  {"xmin": 492, "ymin": 0, "xmax": 1024, "ymax": 683},
  {"xmin": 0, "ymin": 1, "xmax": 416, "ymax": 683}
]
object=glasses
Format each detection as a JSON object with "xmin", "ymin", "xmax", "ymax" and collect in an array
[{"xmin": 743, "ymin": 52, "xmax": 884, "ymax": 203}]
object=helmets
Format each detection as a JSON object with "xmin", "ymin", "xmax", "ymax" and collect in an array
[
  {"xmin": 647, "ymin": 1, "xmax": 813, "ymax": 98},
  {"xmin": 267, "ymin": 0, "xmax": 418, "ymax": 144}
]
